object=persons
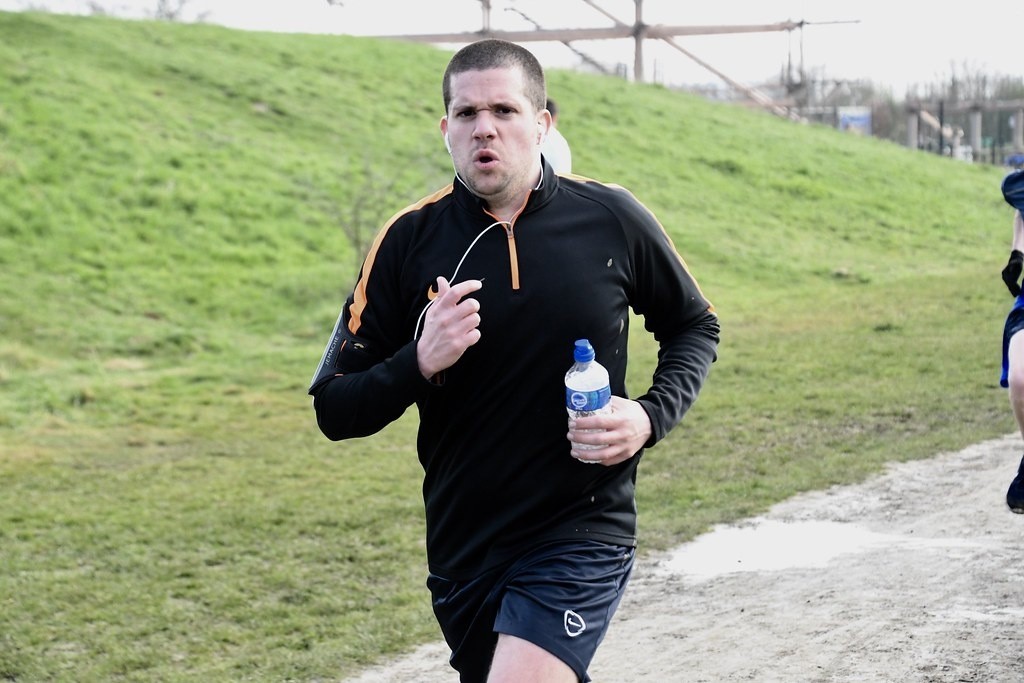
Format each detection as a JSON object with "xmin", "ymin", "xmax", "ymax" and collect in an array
[
  {"xmin": 309, "ymin": 39, "xmax": 721, "ymax": 683},
  {"xmin": 1000, "ymin": 170, "xmax": 1024, "ymax": 514},
  {"xmin": 540, "ymin": 100, "xmax": 572, "ymax": 176}
]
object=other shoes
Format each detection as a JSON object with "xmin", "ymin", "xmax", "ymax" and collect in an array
[{"xmin": 1007, "ymin": 454, "xmax": 1024, "ymax": 514}]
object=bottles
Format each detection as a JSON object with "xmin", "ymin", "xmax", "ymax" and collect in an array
[{"xmin": 564, "ymin": 339, "xmax": 612, "ymax": 464}]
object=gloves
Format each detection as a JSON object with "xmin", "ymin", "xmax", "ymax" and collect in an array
[{"xmin": 1001, "ymin": 250, "xmax": 1024, "ymax": 297}]
